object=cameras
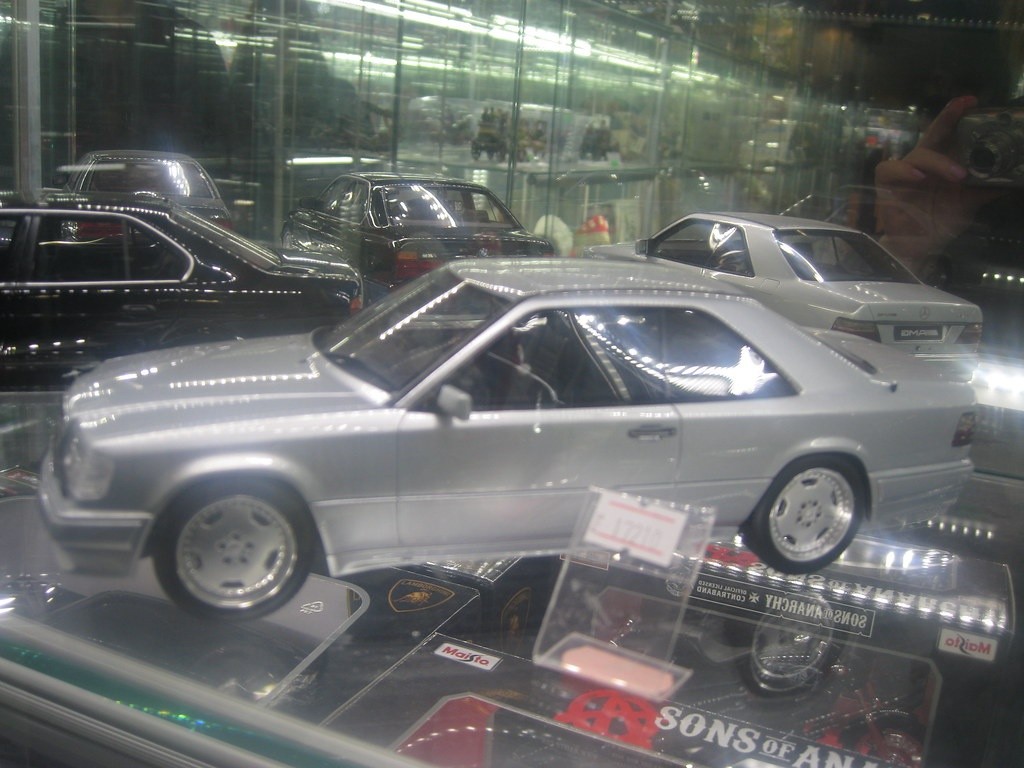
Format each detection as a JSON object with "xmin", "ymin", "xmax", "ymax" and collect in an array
[{"xmin": 957, "ymin": 106, "xmax": 1024, "ymax": 188}]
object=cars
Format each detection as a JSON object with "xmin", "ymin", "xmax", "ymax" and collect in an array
[
  {"xmin": 404, "ymin": 89, "xmax": 927, "ymax": 186},
  {"xmin": 35, "ymin": 257, "xmax": 988, "ymax": 630},
  {"xmin": 581, "ymin": 210, "xmax": 987, "ymax": 373},
  {"xmin": 0, "ymin": 187, "xmax": 367, "ymax": 383},
  {"xmin": 707, "ymin": 183, "xmax": 979, "ymax": 288},
  {"xmin": 54, "ymin": 148, "xmax": 237, "ymax": 235},
  {"xmin": 279, "ymin": 170, "xmax": 560, "ymax": 311}
]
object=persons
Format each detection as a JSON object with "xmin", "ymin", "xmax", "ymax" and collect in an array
[{"xmin": 873, "ymin": 86, "xmax": 988, "ymax": 280}]
